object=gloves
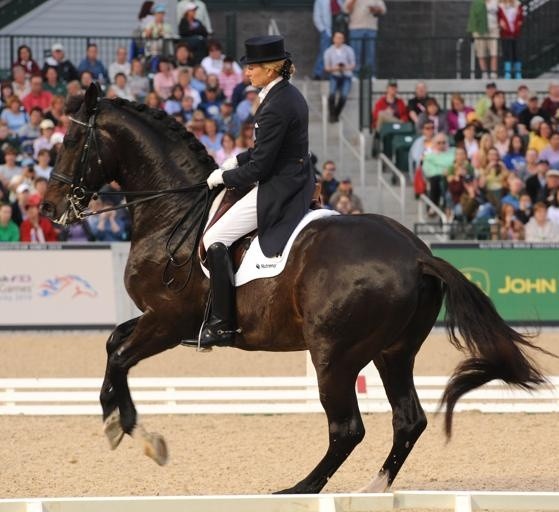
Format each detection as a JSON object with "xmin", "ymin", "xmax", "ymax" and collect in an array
[
  {"xmin": 207, "ymin": 169, "xmax": 224, "ymax": 190},
  {"xmin": 221, "ymin": 157, "xmax": 239, "ymax": 171}
]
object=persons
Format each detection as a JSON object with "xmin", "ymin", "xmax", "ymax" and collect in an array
[
  {"xmin": 465, "ymin": 1, "xmax": 500, "ymax": 78},
  {"xmin": 178, "ymin": 35, "xmax": 317, "ymax": 349},
  {"xmin": 176, "ymin": 1, "xmax": 213, "ymax": 34},
  {"xmin": 1, "ymin": 42, "xmax": 258, "ymax": 241},
  {"xmin": 497, "ymin": 1, "xmax": 528, "ymax": 80},
  {"xmin": 375, "ymin": 79, "xmax": 559, "ymax": 242},
  {"xmin": 320, "ymin": 161, "xmax": 362, "ymax": 214},
  {"xmin": 311, "ymin": 1, "xmax": 350, "ymax": 81},
  {"xmin": 323, "ymin": 30, "xmax": 357, "ymax": 123},
  {"xmin": 138, "ymin": 1, "xmax": 155, "ymax": 31},
  {"xmin": 342, "ymin": 0, "xmax": 388, "ymax": 80},
  {"xmin": 178, "ymin": 4, "xmax": 207, "ymax": 39},
  {"xmin": 140, "ymin": 4, "xmax": 176, "ymax": 56}
]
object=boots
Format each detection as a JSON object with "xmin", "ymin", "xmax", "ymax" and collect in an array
[
  {"xmin": 329, "ymin": 95, "xmax": 345, "ymax": 123},
  {"xmin": 504, "ymin": 61, "xmax": 512, "ymax": 80},
  {"xmin": 181, "ymin": 242, "xmax": 236, "ymax": 348},
  {"xmin": 514, "ymin": 62, "xmax": 522, "ymax": 79}
]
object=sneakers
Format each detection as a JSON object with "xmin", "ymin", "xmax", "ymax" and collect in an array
[{"xmin": 481, "ymin": 72, "xmax": 498, "ymax": 79}]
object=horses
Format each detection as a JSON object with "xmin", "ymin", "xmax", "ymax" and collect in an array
[{"xmin": 34, "ymin": 78, "xmax": 559, "ymax": 494}]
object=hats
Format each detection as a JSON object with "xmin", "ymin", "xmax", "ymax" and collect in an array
[
  {"xmin": 187, "ymin": 3, "xmax": 197, "ymax": 11},
  {"xmin": 21, "ymin": 158, "xmax": 36, "ymax": 166},
  {"xmin": 50, "ymin": 132, "xmax": 64, "ymax": 145},
  {"xmin": 546, "ymin": 169, "xmax": 559, "ymax": 177},
  {"xmin": 40, "ymin": 119, "xmax": 55, "ymax": 130},
  {"xmin": 51, "ymin": 44, "xmax": 62, "ymax": 52},
  {"xmin": 150, "ymin": 3, "xmax": 166, "ymax": 14},
  {"xmin": 16, "ymin": 184, "xmax": 29, "ymax": 193},
  {"xmin": 239, "ymin": 36, "xmax": 287, "ymax": 64},
  {"xmin": 242, "ymin": 85, "xmax": 259, "ymax": 96},
  {"xmin": 24, "ymin": 195, "xmax": 41, "ymax": 210}
]
